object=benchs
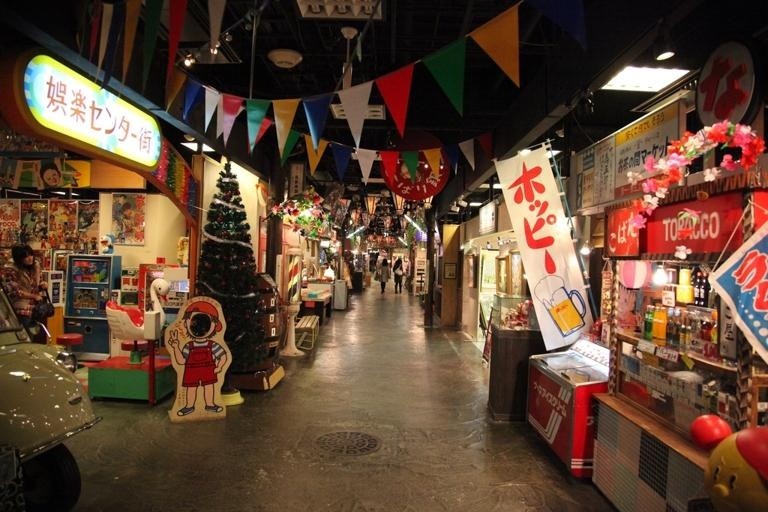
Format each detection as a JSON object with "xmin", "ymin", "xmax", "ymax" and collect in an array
[{"xmin": 294, "ymin": 314, "xmax": 320, "ymax": 350}]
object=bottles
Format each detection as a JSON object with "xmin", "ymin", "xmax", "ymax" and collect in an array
[
  {"xmin": 661, "ymin": 268, "xmax": 711, "ymax": 308},
  {"xmin": 645, "ymin": 304, "xmax": 722, "ymax": 359}
]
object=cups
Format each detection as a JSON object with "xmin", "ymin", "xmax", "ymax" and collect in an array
[{"xmin": 539, "ymin": 287, "xmax": 588, "ymax": 337}]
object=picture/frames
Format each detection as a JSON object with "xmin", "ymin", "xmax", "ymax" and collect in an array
[{"xmin": 444, "ymin": 262, "xmax": 457, "ymax": 279}]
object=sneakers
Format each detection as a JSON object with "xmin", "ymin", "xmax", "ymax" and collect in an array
[
  {"xmin": 177, "ymin": 407, "xmax": 194, "ymax": 416},
  {"xmin": 205, "ymin": 406, "xmax": 223, "ymax": 412}
]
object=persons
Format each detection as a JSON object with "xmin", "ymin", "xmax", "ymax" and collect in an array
[
  {"xmin": 375, "ymin": 258, "xmax": 391, "ymax": 295},
  {"xmin": 6, "ymin": 243, "xmax": 54, "ymax": 348},
  {"xmin": 392, "ymin": 258, "xmax": 403, "ymax": 294}
]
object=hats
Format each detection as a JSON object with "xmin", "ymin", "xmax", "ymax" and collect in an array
[{"xmin": 185, "ymin": 301, "xmax": 222, "ymax": 332}]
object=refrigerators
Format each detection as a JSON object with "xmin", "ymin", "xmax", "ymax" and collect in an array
[{"xmin": 524, "ymin": 347, "xmax": 614, "ymax": 478}]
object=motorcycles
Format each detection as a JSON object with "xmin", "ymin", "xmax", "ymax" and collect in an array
[{"xmin": 0, "ymin": 286, "xmax": 101, "ymax": 505}]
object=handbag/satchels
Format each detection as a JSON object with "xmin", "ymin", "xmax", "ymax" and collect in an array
[
  {"xmin": 374, "ymin": 270, "xmax": 382, "ymax": 282},
  {"xmin": 32, "ymin": 304, "xmax": 55, "ymax": 319},
  {"xmin": 394, "ymin": 268, "xmax": 402, "ymax": 275}
]
